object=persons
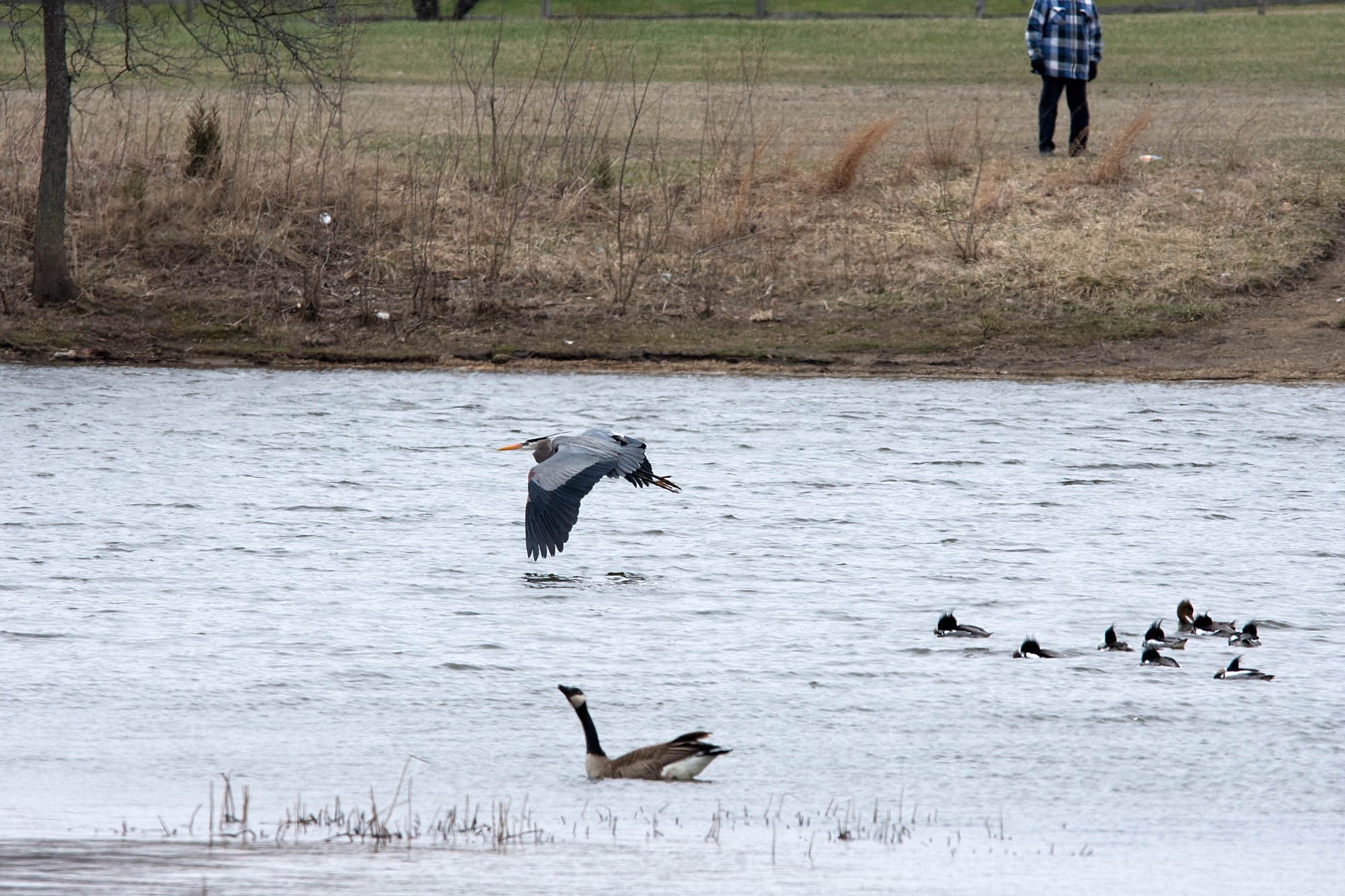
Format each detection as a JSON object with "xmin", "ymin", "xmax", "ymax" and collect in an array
[{"xmin": 1024, "ymin": 0, "xmax": 1103, "ymax": 157}]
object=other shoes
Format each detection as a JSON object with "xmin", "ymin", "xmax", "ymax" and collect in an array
[
  {"xmin": 1039, "ymin": 151, "xmax": 1055, "ymax": 159},
  {"xmin": 1074, "ymin": 150, "xmax": 1097, "ymax": 158}
]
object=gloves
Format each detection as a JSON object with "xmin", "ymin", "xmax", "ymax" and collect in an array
[
  {"xmin": 1088, "ymin": 61, "xmax": 1097, "ymax": 82},
  {"xmin": 1030, "ymin": 59, "xmax": 1046, "ymax": 81}
]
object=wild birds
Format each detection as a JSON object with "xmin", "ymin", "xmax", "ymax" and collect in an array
[
  {"xmin": 496, "ymin": 429, "xmax": 683, "ymax": 563},
  {"xmin": 559, "ymin": 682, "xmax": 733, "ymax": 781},
  {"xmin": 933, "ymin": 609, "xmax": 993, "ymax": 638},
  {"xmin": 1013, "ymin": 599, "xmax": 1277, "ymax": 681}
]
